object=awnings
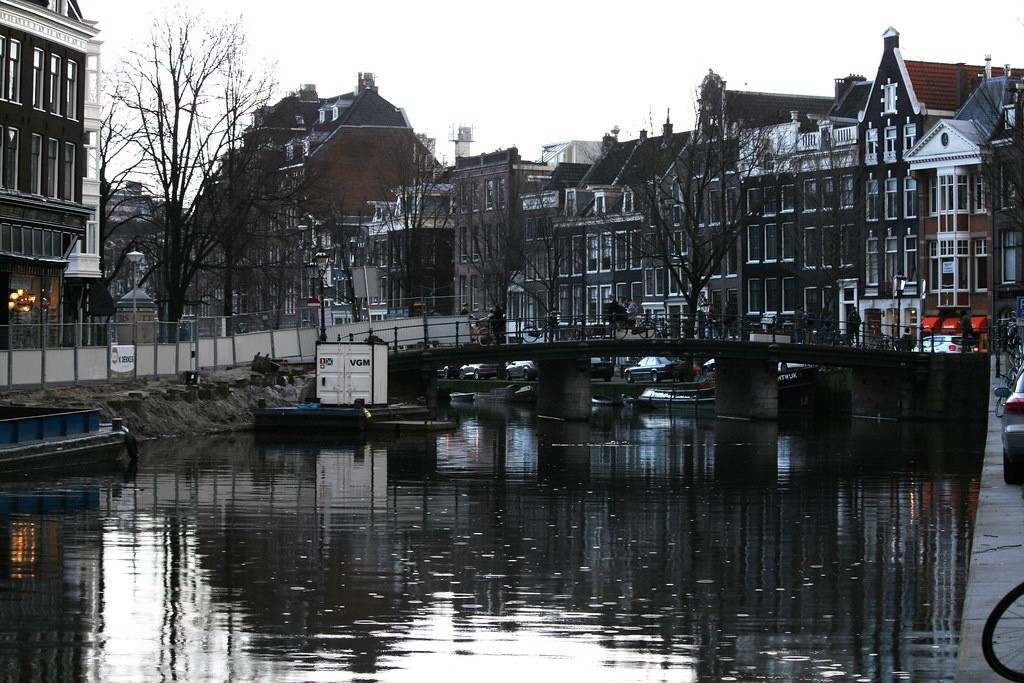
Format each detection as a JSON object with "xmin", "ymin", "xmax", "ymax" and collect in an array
[
  {"xmin": 88, "ymin": 280, "xmax": 114, "ymax": 316},
  {"xmin": 921, "ymin": 315, "xmax": 987, "ymax": 334}
]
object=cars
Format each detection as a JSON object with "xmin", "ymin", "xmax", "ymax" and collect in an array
[
  {"xmin": 459, "ymin": 364, "xmax": 499, "ymax": 381},
  {"xmin": 911, "ymin": 335, "xmax": 978, "ymax": 354},
  {"xmin": 438, "ymin": 365, "xmax": 459, "ymax": 379},
  {"xmin": 590, "ymin": 358, "xmax": 614, "ymax": 381},
  {"xmin": 994, "ymin": 362, "xmax": 1024, "ymax": 484},
  {"xmin": 623, "ymin": 357, "xmax": 685, "ymax": 384},
  {"xmin": 504, "ymin": 360, "xmax": 540, "ymax": 380}
]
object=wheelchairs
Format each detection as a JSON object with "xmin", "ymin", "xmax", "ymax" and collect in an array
[{"xmin": 472, "ymin": 321, "xmax": 496, "ymax": 345}]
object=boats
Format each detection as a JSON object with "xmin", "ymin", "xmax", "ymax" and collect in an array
[
  {"xmin": 501, "ymin": 384, "xmax": 536, "ymax": 401},
  {"xmin": 450, "ymin": 389, "xmax": 499, "ymax": 401},
  {"xmin": 592, "ymin": 396, "xmax": 617, "ymax": 405},
  {"xmin": 621, "ymin": 366, "xmax": 818, "ymax": 408},
  {"xmin": 0, "ymin": 403, "xmax": 128, "ymax": 471}
]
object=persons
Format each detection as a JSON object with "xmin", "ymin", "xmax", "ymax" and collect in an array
[
  {"xmin": 607, "ymin": 294, "xmax": 638, "ymax": 334},
  {"xmin": 901, "ymin": 327, "xmax": 913, "ymax": 351},
  {"xmin": 958, "ymin": 310, "xmax": 972, "ymax": 353},
  {"xmin": 848, "ymin": 306, "xmax": 862, "ymax": 348},
  {"xmin": 706, "ymin": 301, "xmax": 737, "ymax": 340},
  {"xmin": 461, "ymin": 302, "xmax": 507, "ymax": 345},
  {"xmin": 794, "ymin": 305, "xmax": 837, "ymax": 346}
]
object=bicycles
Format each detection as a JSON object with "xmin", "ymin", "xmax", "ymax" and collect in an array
[
  {"xmin": 994, "ymin": 324, "xmax": 1024, "ymax": 418},
  {"xmin": 589, "ymin": 315, "xmax": 721, "ymax": 341},
  {"xmin": 522, "ymin": 320, "xmax": 564, "ymax": 343},
  {"xmin": 982, "ymin": 582, "xmax": 1024, "ymax": 683}
]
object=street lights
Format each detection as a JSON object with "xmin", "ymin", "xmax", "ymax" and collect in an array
[
  {"xmin": 894, "ymin": 272, "xmax": 906, "ymax": 339},
  {"xmin": 126, "ymin": 251, "xmax": 144, "ymax": 379},
  {"xmin": 306, "ymin": 248, "xmax": 328, "ymax": 342}
]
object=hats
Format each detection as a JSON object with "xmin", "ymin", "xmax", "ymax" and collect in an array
[{"xmin": 608, "ymin": 294, "xmax": 616, "ymax": 298}]
